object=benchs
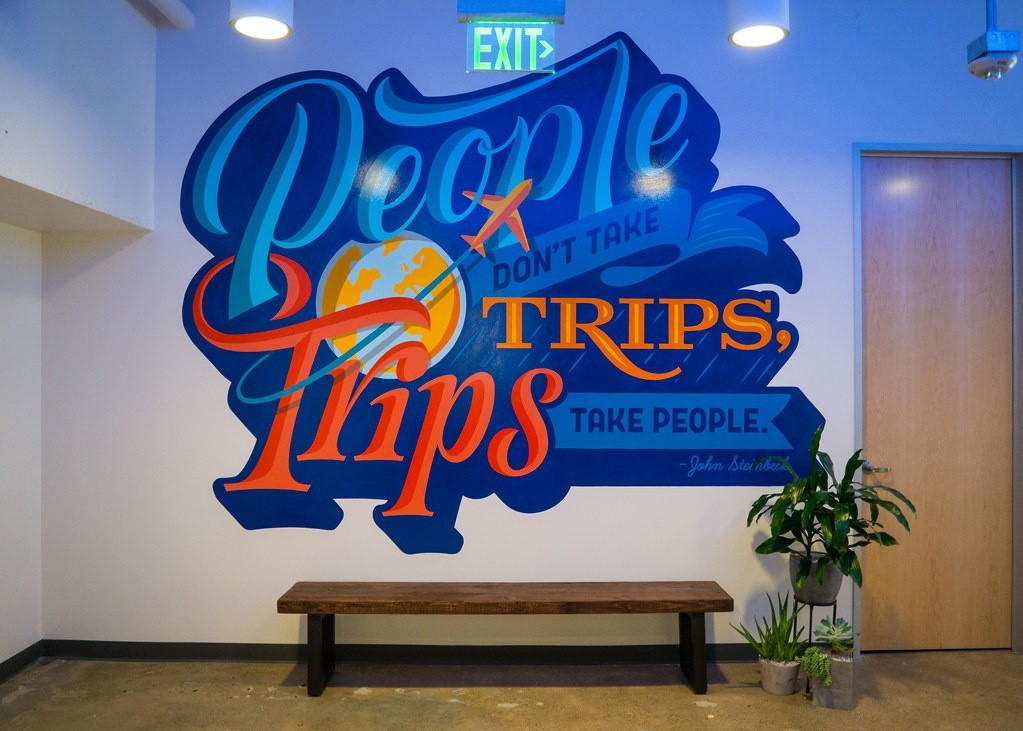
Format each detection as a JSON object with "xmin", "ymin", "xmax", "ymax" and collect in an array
[{"xmin": 277, "ymin": 580, "xmax": 735, "ymax": 697}]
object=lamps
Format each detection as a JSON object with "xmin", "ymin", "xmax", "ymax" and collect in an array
[
  {"xmin": 727, "ymin": 0, "xmax": 790, "ymax": 48},
  {"xmin": 229, "ymin": 0, "xmax": 294, "ymax": 41}
]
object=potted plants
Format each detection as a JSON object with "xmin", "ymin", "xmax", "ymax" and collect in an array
[
  {"xmin": 729, "ymin": 588, "xmax": 811, "ymax": 696},
  {"xmin": 801, "ymin": 616, "xmax": 861, "ymax": 712},
  {"xmin": 746, "ymin": 419, "xmax": 917, "ymax": 603}
]
